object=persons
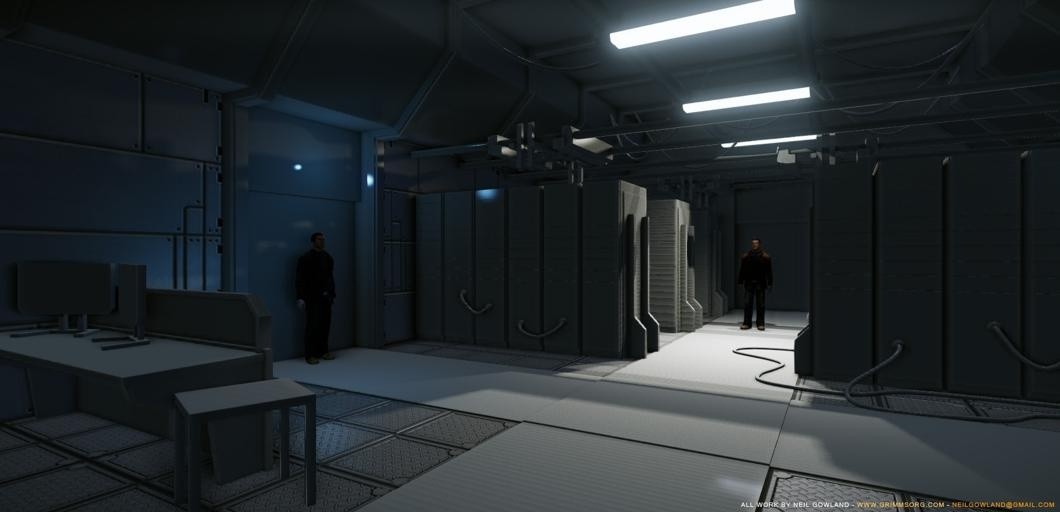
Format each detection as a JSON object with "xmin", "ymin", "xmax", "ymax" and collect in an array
[
  {"xmin": 296, "ymin": 233, "xmax": 336, "ymax": 364},
  {"xmin": 737, "ymin": 239, "xmax": 773, "ymax": 330}
]
